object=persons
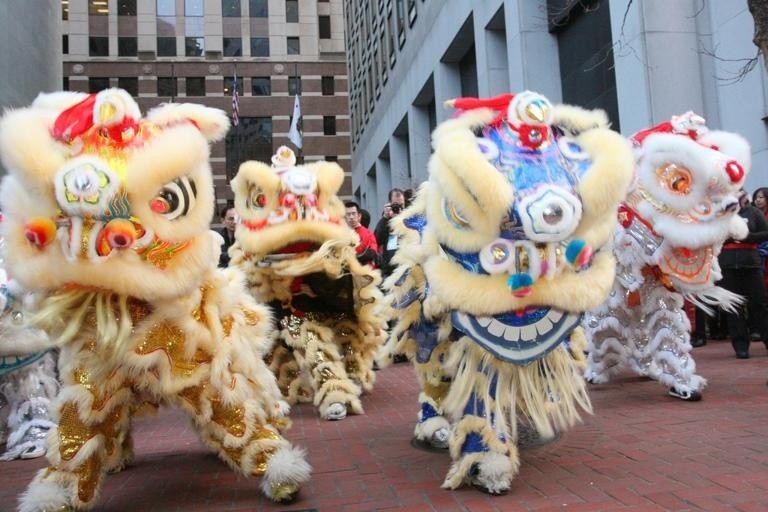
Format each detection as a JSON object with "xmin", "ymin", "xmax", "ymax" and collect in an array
[
  {"xmin": 358, "ymin": 209, "xmax": 371, "ymax": 230},
  {"xmin": 346, "ymin": 201, "xmax": 385, "ymax": 371},
  {"xmin": 683, "ymin": 187, "xmax": 767, "ymax": 357},
  {"xmin": 373, "ymin": 187, "xmax": 410, "ymax": 363},
  {"xmin": 218, "ymin": 203, "xmax": 236, "ymax": 267}
]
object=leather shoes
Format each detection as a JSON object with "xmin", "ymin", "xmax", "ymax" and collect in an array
[
  {"xmin": 736, "ymin": 351, "xmax": 749, "ymax": 358},
  {"xmin": 692, "ymin": 337, "xmax": 707, "ymax": 347}
]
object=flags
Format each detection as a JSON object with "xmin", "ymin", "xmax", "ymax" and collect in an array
[
  {"xmin": 288, "ymin": 94, "xmax": 304, "ymax": 151},
  {"xmin": 232, "ymin": 74, "xmax": 240, "ymax": 127}
]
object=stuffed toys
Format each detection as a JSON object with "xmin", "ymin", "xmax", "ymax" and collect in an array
[
  {"xmin": 0, "ymin": 180, "xmax": 65, "ymax": 463},
  {"xmin": 221, "ymin": 147, "xmax": 391, "ymax": 422},
  {"xmin": 0, "ymin": 88, "xmax": 316, "ymax": 510},
  {"xmin": 387, "ymin": 88, "xmax": 635, "ymax": 493},
  {"xmin": 578, "ymin": 110, "xmax": 750, "ymax": 399}
]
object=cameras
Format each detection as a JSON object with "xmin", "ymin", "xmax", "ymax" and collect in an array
[{"xmin": 391, "ymin": 202, "xmax": 403, "ymax": 214}]
